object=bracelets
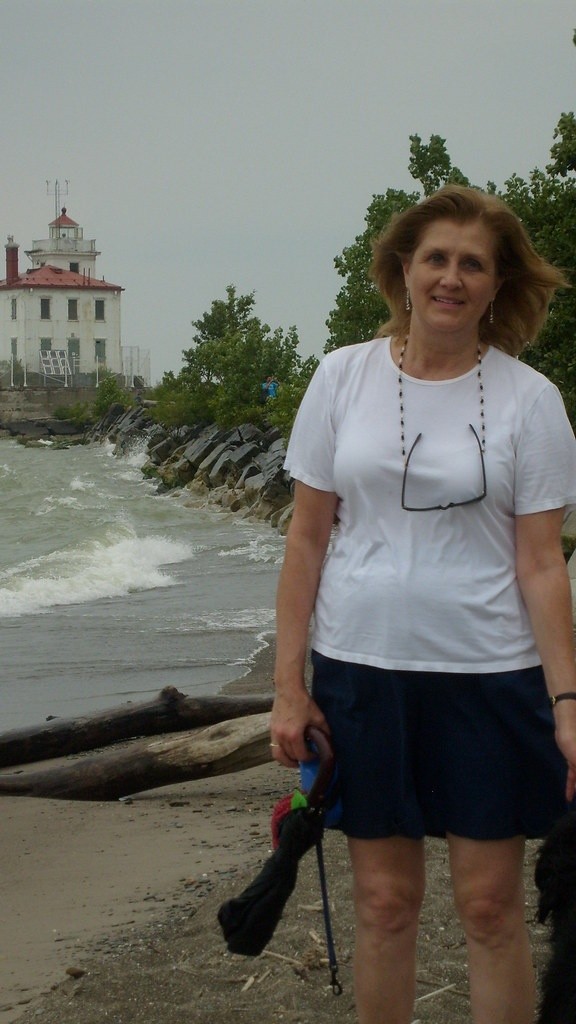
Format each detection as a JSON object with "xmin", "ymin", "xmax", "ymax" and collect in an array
[{"xmin": 549, "ymin": 692, "xmax": 576, "ymax": 709}]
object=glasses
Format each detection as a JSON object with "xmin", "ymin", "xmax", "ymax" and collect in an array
[{"xmin": 401, "ymin": 424, "xmax": 486, "ymax": 511}]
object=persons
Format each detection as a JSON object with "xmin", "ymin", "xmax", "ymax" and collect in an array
[
  {"xmin": 271, "ymin": 186, "xmax": 576, "ymax": 1024},
  {"xmin": 261, "ymin": 376, "xmax": 279, "ymax": 405}
]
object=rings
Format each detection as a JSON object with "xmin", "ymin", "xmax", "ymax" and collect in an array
[{"xmin": 271, "ymin": 743, "xmax": 281, "ymax": 747}]
